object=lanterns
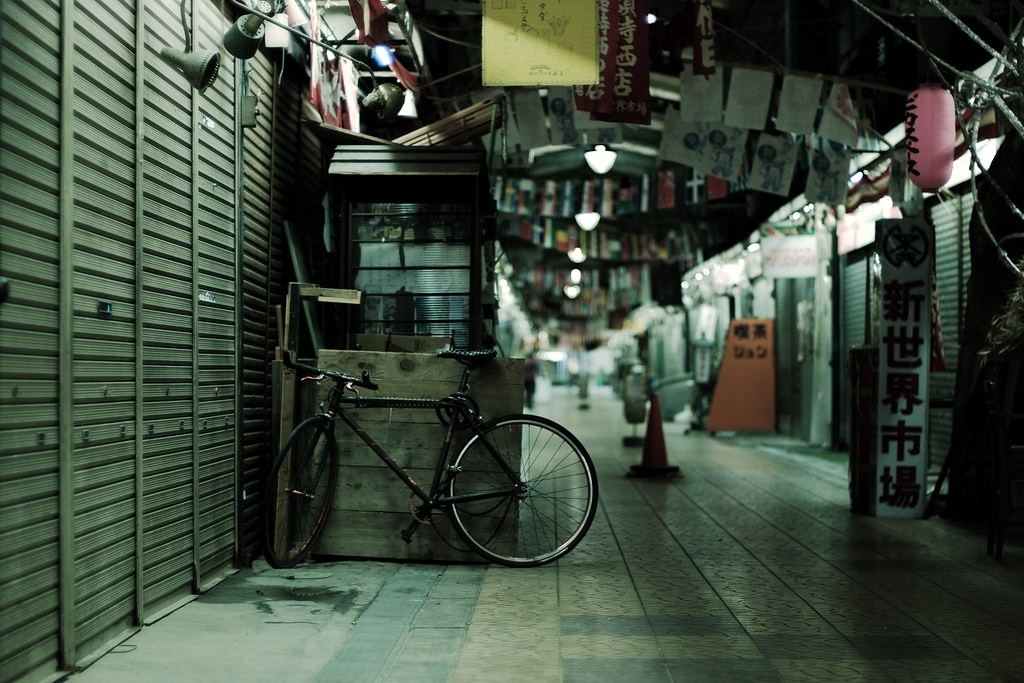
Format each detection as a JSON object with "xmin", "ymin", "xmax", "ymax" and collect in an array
[{"xmin": 903, "ymin": 82, "xmax": 956, "ymax": 194}]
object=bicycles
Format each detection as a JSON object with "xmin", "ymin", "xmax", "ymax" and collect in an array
[{"xmin": 262, "ymin": 342, "xmax": 599, "ymax": 570}]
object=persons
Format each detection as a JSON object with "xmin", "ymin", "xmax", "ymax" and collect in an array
[{"xmin": 524, "ymin": 354, "xmax": 540, "ymax": 409}]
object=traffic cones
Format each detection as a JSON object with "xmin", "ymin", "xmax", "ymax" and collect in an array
[{"xmin": 625, "ymin": 393, "xmax": 681, "ymax": 478}]
